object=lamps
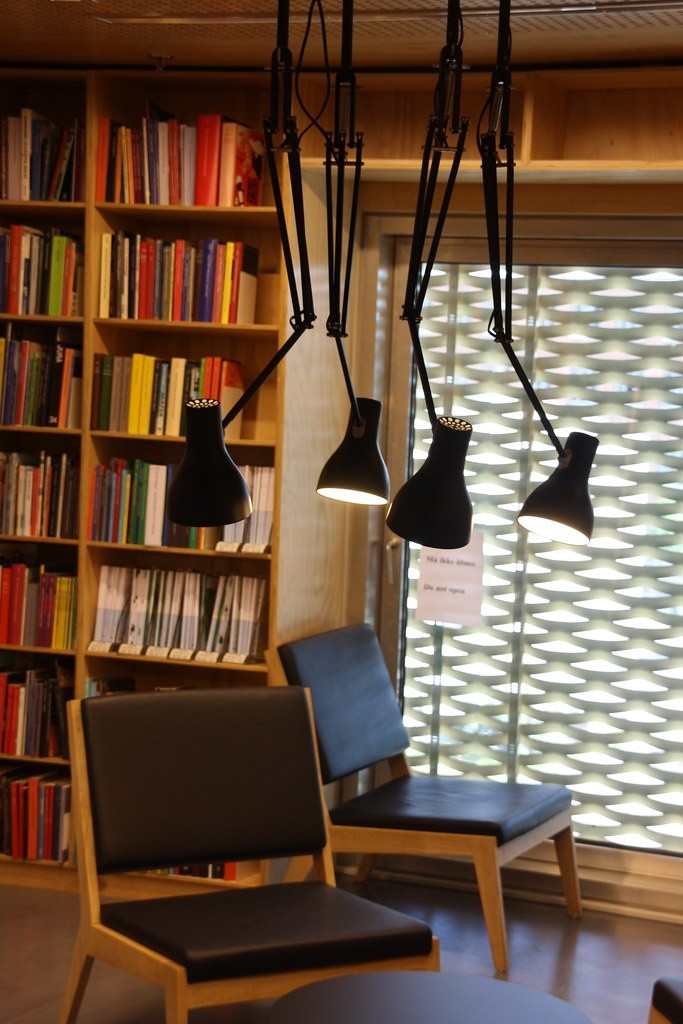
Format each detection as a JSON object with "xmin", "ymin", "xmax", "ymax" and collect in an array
[{"xmin": 171, "ymin": 0, "xmax": 602, "ymax": 547}]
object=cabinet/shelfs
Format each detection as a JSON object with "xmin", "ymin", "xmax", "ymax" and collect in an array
[{"xmin": 1, "ymin": 70, "xmax": 350, "ymax": 891}]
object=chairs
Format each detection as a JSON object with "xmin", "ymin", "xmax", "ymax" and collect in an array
[
  {"xmin": 278, "ymin": 622, "xmax": 582, "ymax": 969},
  {"xmin": 48, "ymin": 686, "xmax": 441, "ymax": 1024}
]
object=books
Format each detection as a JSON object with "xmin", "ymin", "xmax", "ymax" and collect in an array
[
  {"xmin": 0, "ymin": 448, "xmax": 80, "ymax": 538},
  {"xmin": 99, "ymin": 229, "xmax": 259, "ymax": 325},
  {"xmin": 0, "ymin": 223, "xmax": 84, "ymax": 317},
  {"xmin": 85, "ymin": 674, "xmax": 236, "ymax": 881},
  {"xmin": 0, "ymin": 320, "xmax": 83, "ymax": 430},
  {"xmin": 0, "ymin": 554, "xmax": 78, "ymax": 650},
  {"xmin": 0, "ymin": 106, "xmax": 87, "ymax": 203},
  {"xmin": 86, "ymin": 456, "xmax": 276, "ymax": 554},
  {"xmin": 96, "ymin": 99, "xmax": 266, "ymax": 208},
  {"xmin": 90, "ymin": 353, "xmax": 245, "ymax": 439},
  {"xmin": 1, "ymin": 761, "xmax": 76, "ymax": 868},
  {"xmin": 93, "ymin": 565, "xmax": 269, "ymax": 656},
  {"xmin": 0, "ymin": 654, "xmax": 73, "ymax": 760}
]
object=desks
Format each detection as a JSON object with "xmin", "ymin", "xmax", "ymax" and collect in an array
[{"xmin": 266, "ymin": 971, "xmax": 596, "ymax": 1024}]
